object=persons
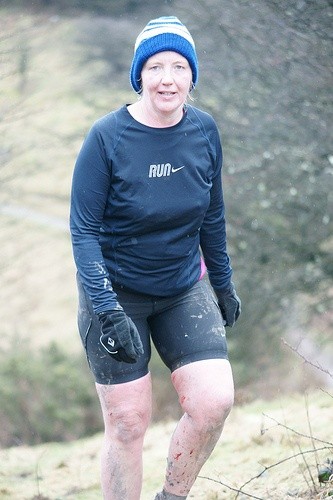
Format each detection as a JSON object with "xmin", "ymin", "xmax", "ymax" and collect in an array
[{"xmin": 69, "ymin": 15, "xmax": 243, "ymax": 500}]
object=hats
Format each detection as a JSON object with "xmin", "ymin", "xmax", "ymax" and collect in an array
[{"xmin": 129, "ymin": 14, "xmax": 198, "ymax": 96}]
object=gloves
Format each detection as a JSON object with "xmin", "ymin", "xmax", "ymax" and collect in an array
[
  {"xmin": 213, "ymin": 281, "xmax": 242, "ymax": 328},
  {"xmin": 97, "ymin": 310, "xmax": 144, "ymax": 364}
]
